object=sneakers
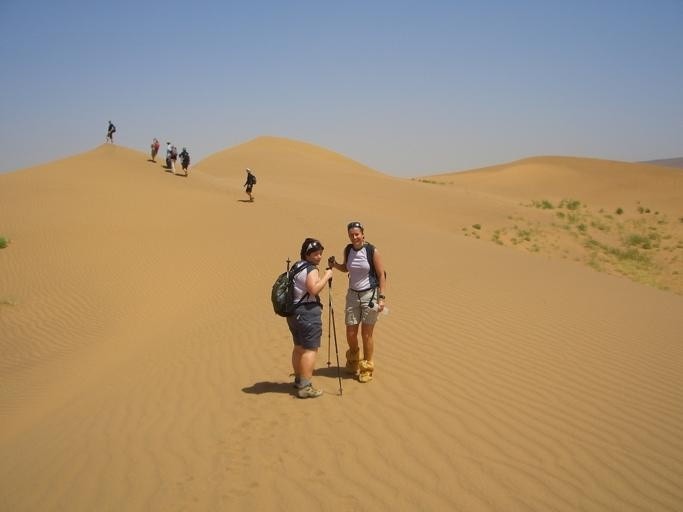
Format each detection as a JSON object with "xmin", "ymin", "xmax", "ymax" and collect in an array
[
  {"xmin": 170, "ymin": 168, "xmax": 176, "ymax": 173},
  {"xmin": 248, "ymin": 196, "xmax": 255, "ymax": 202},
  {"xmin": 185, "ymin": 170, "xmax": 189, "ymax": 177},
  {"xmin": 292, "ymin": 382, "xmax": 323, "ymax": 398}
]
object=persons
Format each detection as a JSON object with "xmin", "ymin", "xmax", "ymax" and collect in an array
[
  {"xmin": 155, "ymin": 139, "xmax": 159, "ymax": 153},
  {"xmin": 327, "ymin": 221, "xmax": 386, "ymax": 383},
  {"xmin": 104, "ymin": 120, "xmax": 115, "ymax": 144},
  {"xmin": 242, "ymin": 167, "xmax": 256, "ymax": 202},
  {"xmin": 164, "ymin": 141, "xmax": 171, "ymax": 170},
  {"xmin": 178, "ymin": 146, "xmax": 190, "ymax": 177},
  {"xmin": 285, "ymin": 238, "xmax": 332, "ymax": 398},
  {"xmin": 150, "ymin": 138, "xmax": 156, "ymax": 162},
  {"xmin": 169, "ymin": 145, "xmax": 177, "ymax": 174}
]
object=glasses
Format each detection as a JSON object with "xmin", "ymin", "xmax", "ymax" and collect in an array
[
  {"xmin": 305, "ymin": 241, "xmax": 321, "ymax": 255},
  {"xmin": 348, "ymin": 222, "xmax": 363, "ymax": 232}
]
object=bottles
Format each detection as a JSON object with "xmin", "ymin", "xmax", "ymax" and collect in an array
[{"xmin": 368, "ymin": 302, "xmax": 388, "ymax": 316}]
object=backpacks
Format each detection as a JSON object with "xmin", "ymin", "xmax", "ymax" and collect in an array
[
  {"xmin": 182, "ymin": 152, "xmax": 190, "ymax": 165},
  {"xmin": 271, "ymin": 262, "xmax": 316, "ymax": 317},
  {"xmin": 345, "ymin": 244, "xmax": 387, "ymax": 282},
  {"xmin": 154, "ymin": 142, "xmax": 160, "ymax": 150},
  {"xmin": 249, "ymin": 174, "xmax": 257, "ymax": 185},
  {"xmin": 112, "ymin": 124, "xmax": 116, "ymax": 132},
  {"xmin": 171, "ymin": 148, "xmax": 177, "ymax": 159}
]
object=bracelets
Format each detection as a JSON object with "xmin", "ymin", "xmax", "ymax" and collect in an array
[{"xmin": 377, "ymin": 294, "xmax": 385, "ymax": 299}]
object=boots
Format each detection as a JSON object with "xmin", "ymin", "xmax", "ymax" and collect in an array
[
  {"xmin": 344, "ymin": 347, "xmax": 360, "ymax": 375},
  {"xmin": 359, "ymin": 360, "xmax": 374, "ymax": 382}
]
